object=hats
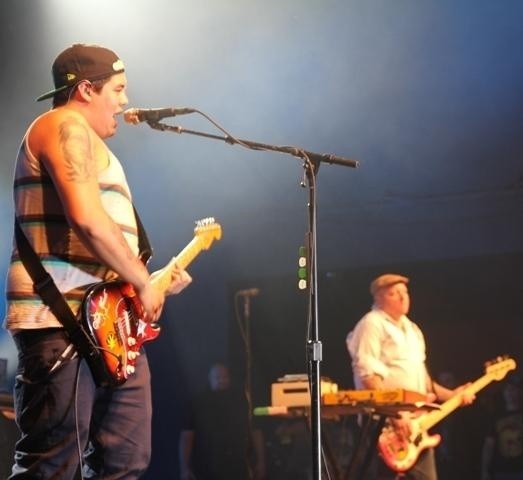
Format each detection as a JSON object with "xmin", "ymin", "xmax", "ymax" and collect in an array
[
  {"xmin": 34, "ymin": 41, "xmax": 126, "ymax": 103},
  {"xmin": 367, "ymin": 272, "xmax": 409, "ymax": 296}
]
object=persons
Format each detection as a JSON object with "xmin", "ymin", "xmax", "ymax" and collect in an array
[
  {"xmin": 0, "ymin": 41, "xmax": 193, "ymax": 480},
  {"xmin": 343, "ymin": 272, "xmax": 476, "ymax": 480},
  {"xmin": 477, "ymin": 378, "xmax": 523, "ymax": 479},
  {"xmin": 175, "ymin": 360, "xmax": 268, "ymax": 480}
]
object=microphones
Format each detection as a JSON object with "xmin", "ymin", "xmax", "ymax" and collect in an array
[
  {"xmin": 237, "ymin": 285, "xmax": 259, "ymax": 300},
  {"xmin": 122, "ymin": 106, "xmax": 197, "ymax": 128}
]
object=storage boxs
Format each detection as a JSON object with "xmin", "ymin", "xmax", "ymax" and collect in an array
[{"xmin": 271, "ymin": 378, "xmax": 338, "ymax": 407}]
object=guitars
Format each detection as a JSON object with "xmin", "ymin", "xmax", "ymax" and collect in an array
[
  {"xmin": 81, "ymin": 216, "xmax": 222, "ymax": 386},
  {"xmin": 378, "ymin": 354, "xmax": 517, "ymax": 473}
]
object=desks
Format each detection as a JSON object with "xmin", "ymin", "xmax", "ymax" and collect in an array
[{"xmin": 254, "ymin": 404, "xmax": 441, "ymax": 480}]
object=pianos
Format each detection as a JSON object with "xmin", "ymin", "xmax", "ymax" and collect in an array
[{"xmin": 322, "ymin": 390, "xmax": 429, "ymax": 407}]
